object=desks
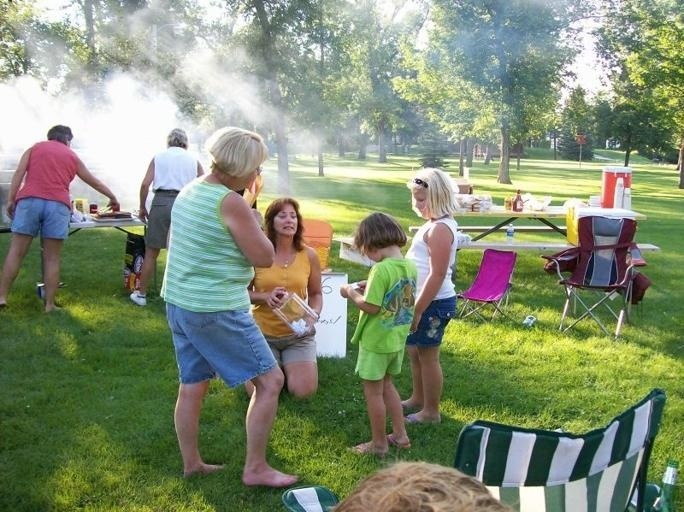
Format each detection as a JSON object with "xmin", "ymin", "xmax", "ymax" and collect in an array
[
  {"xmin": 463, "ymin": 205, "xmax": 647, "ymax": 242},
  {"xmin": 69, "ymin": 212, "xmax": 160, "ymax": 297}
]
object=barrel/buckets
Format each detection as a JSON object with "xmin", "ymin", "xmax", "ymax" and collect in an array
[{"xmin": 601, "ymin": 166, "xmax": 632, "ymax": 208}]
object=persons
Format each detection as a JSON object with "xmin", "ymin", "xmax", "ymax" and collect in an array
[
  {"xmin": 340, "ymin": 212, "xmax": 419, "ymax": 458},
  {"xmin": 245, "ymin": 198, "xmax": 323, "ymax": 400},
  {"xmin": 160, "ymin": 126, "xmax": 300, "ymax": 488},
  {"xmin": 403, "ymin": 167, "xmax": 460, "ymax": 425},
  {"xmin": 129, "ymin": 129, "xmax": 204, "ymax": 307},
  {"xmin": 331, "ymin": 461, "xmax": 512, "ymax": 512},
  {"xmin": 0, "ymin": 125, "xmax": 120, "ymax": 314}
]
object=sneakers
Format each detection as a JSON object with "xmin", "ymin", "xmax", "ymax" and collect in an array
[{"xmin": 129, "ymin": 291, "xmax": 147, "ymax": 306}]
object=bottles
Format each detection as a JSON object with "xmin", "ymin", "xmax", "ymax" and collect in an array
[
  {"xmin": 504, "ymin": 195, "xmax": 512, "ymax": 209},
  {"xmin": 272, "ymin": 290, "xmax": 319, "ymax": 337},
  {"xmin": 649, "ymin": 460, "xmax": 679, "ymax": 512},
  {"xmin": 506, "ymin": 224, "xmax": 515, "ymax": 244},
  {"xmin": 513, "ymin": 189, "xmax": 524, "ymax": 212},
  {"xmin": 522, "ymin": 315, "xmax": 537, "ymax": 327}
]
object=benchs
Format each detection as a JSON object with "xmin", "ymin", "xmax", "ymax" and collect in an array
[
  {"xmin": 458, "ymin": 242, "xmax": 661, "ymax": 250},
  {"xmin": 408, "ymin": 225, "xmax": 569, "ymax": 233}
]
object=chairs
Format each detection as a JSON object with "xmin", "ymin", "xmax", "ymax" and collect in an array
[
  {"xmin": 301, "ymin": 220, "xmax": 332, "ymax": 272},
  {"xmin": 459, "ymin": 249, "xmax": 518, "ymax": 325},
  {"xmin": 282, "ymin": 389, "xmax": 667, "ymax": 512},
  {"xmin": 541, "ymin": 217, "xmax": 652, "ymax": 336}
]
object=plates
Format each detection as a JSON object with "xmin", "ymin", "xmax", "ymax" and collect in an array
[{"xmin": 589, "ymin": 196, "xmax": 601, "ymax": 207}]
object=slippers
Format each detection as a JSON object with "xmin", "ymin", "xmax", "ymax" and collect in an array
[
  {"xmin": 387, "ymin": 433, "xmax": 411, "ymax": 449},
  {"xmin": 401, "ymin": 400, "xmax": 424, "ymax": 410},
  {"xmin": 353, "ymin": 441, "xmax": 388, "ymax": 458},
  {"xmin": 406, "ymin": 412, "xmax": 442, "ymax": 425}
]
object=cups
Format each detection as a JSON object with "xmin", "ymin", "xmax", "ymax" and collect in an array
[{"xmin": 614, "ymin": 178, "xmax": 632, "ymax": 210}]
